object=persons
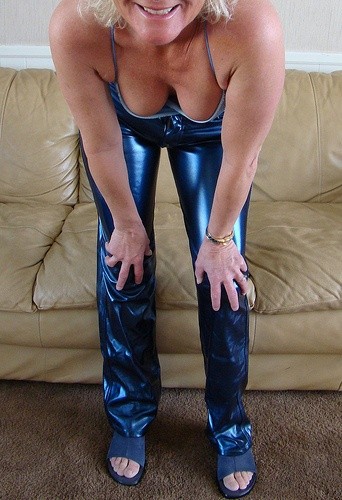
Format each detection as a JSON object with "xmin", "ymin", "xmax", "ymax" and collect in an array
[{"xmin": 49, "ymin": 0, "xmax": 285, "ymax": 499}]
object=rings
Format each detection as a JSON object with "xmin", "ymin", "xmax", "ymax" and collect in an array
[{"xmin": 238, "ymin": 274, "xmax": 247, "ymax": 284}]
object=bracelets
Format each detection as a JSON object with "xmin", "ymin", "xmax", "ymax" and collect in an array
[{"xmin": 206, "ymin": 225, "xmax": 235, "ymax": 246}]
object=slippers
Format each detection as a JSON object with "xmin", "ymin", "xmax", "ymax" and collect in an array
[
  {"xmin": 218, "ymin": 449, "xmax": 257, "ymax": 499},
  {"xmin": 106, "ymin": 431, "xmax": 146, "ymax": 485}
]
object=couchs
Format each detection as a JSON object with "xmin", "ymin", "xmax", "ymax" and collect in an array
[{"xmin": 0, "ymin": 67, "xmax": 342, "ymax": 390}]
